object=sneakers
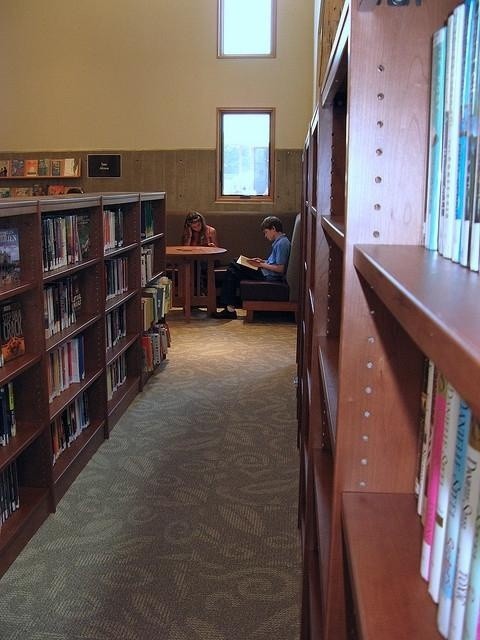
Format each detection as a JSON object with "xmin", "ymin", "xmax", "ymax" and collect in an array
[{"xmin": 212, "ymin": 309, "xmax": 237, "ymax": 319}]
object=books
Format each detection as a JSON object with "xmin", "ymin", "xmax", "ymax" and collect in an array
[
  {"xmin": 236, "ymin": 254, "xmax": 259, "ymax": 271},
  {"xmin": 140, "ymin": 200, "xmax": 173, "ymax": 372},
  {"xmin": 424, "ymin": 0, "xmax": 480, "ymax": 273},
  {"xmin": 102, "ymin": 209, "xmax": 128, "ymax": 399},
  {"xmin": 1, "ymin": 225, "xmax": 26, "ymax": 526},
  {"xmin": 0, "ymin": 158, "xmax": 84, "ymax": 198},
  {"xmin": 43, "ymin": 212, "xmax": 91, "ymax": 467},
  {"xmin": 414, "ymin": 357, "xmax": 480, "ymax": 640}
]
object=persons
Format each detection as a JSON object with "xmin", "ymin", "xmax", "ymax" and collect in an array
[
  {"xmin": 180, "ymin": 211, "xmax": 216, "ymax": 292},
  {"xmin": 217, "ymin": 215, "xmax": 291, "ymax": 319}
]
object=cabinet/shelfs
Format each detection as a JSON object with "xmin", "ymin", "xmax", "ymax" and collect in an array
[
  {"xmin": 295, "ymin": 0, "xmax": 479, "ymax": 639},
  {"xmin": 0, "ymin": 189, "xmax": 168, "ymax": 579}
]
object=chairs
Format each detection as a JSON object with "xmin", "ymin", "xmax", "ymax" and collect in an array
[{"xmin": 239, "ymin": 212, "xmax": 301, "ymax": 323}]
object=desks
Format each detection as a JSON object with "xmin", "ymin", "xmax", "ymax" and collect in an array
[{"xmin": 164, "ymin": 246, "xmax": 228, "ymax": 323}]
